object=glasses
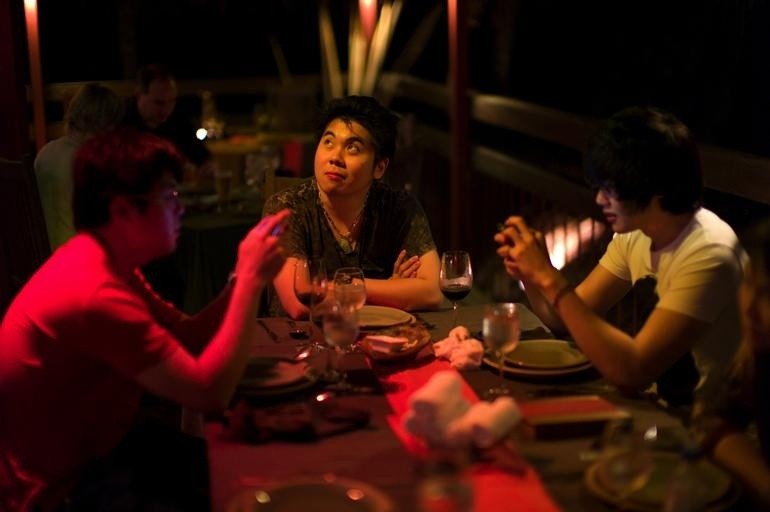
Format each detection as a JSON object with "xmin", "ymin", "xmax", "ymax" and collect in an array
[{"xmin": 137, "ymin": 188, "xmax": 181, "ymax": 211}]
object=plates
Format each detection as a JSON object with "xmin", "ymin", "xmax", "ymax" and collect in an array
[
  {"xmin": 237, "ymin": 357, "xmax": 318, "ymax": 399},
  {"xmin": 488, "ymin": 339, "xmax": 593, "ymax": 381},
  {"xmin": 584, "ymin": 449, "xmax": 738, "ymax": 504},
  {"xmin": 349, "ymin": 304, "xmax": 418, "ymax": 332}
]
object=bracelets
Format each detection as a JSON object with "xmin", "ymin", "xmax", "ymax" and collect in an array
[
  {"xmin": 552, "ymin": 283, "xmax": 572, "ymax": 309},
  {"xmin": 227, "ymin": 272, "xmax": 237, "ymax": 286}
]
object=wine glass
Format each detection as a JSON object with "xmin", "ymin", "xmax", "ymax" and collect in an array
[
  {"xmin": 436, "ymin": 251, "xmax": 474, "ymax": 327},
  {"xmin": 294, "ymin": 257, "xmax": 368, "ymax": 393},
  {"xmin": 482, "ymin": 304, "xmax": 520, "ymax": 396}
]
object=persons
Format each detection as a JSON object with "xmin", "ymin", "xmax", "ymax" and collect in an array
[
  {"xmin": 488, "ymin": 105, "xmax": 751, "ymax": 431},
  {"xmin": 252, "ymin": 94, "xmax": 451, "ymax": 321},
  {"xmin": 672, "ymin": 213, "xmax": 770, "ymax": 510},
  {"xmin": 107, "ymin": 61, "xmax": 180, "ymax": 147},
  {"xmin": 0, "ymin": 127, "xmax": 301, "ymax": 512},
  {"xmin": 33, "ymin": 77, "xmax": 127, "ymax": 253}
]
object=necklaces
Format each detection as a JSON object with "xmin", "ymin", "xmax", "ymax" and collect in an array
[{"xmin": 318, "ymin": 196, "xmax": 366, "ymax": 253}]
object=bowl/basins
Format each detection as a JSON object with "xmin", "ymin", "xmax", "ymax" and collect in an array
[{"xmin": 356, "ymin": 328, "xmax": 432, "ymax": 364}]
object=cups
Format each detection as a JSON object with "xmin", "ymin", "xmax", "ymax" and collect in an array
[{"xmin": 212, "ymin": 165, "xmax": 232, "ymax": 207}]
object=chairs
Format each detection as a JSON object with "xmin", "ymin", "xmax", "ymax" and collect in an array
[{"xmin": 258, "ymin": 167, "xmax": 410, "ymax": 299}]
